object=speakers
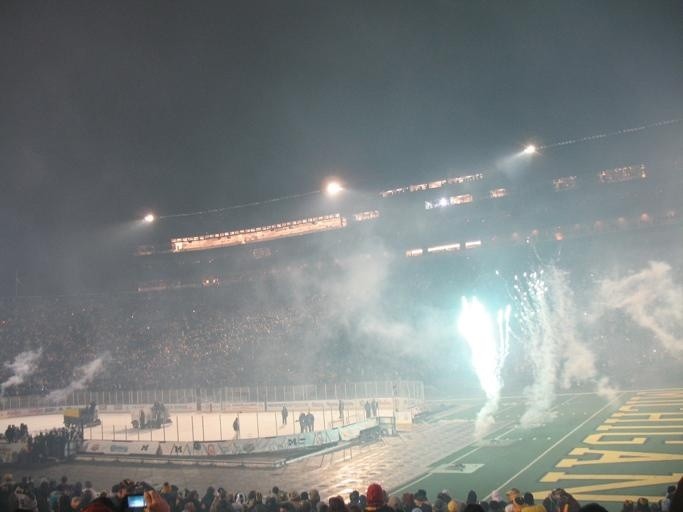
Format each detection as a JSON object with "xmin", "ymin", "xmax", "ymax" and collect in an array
[{"xmin": 359, "ymin": 426, "xmax": 381, "ymax": 441}]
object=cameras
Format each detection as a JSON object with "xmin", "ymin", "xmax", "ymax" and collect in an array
[{"xmin": 122, "ymin": 494, "xmax": 147, "ymax": 510}]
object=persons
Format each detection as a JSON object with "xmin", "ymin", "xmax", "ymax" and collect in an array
[
  {"xmin": 620, "ymin": 478, "xmax": 683, "ymax": 512},
  {"xmin": 233, "ymin": 418, "xmax": 239, "ymax": 440},
  {"xmin": 449, "ymin": 488, "xmax": 608, "ymax": 512},
  {"xmin": 0, "ymin": 474, "xmax": 201, "ymax": 512},
  {"xmin": 339, "ymin": 400, "xmax": 345, "ymax": 419},
  {"xmin": 0, "ymin": 268, "xmax": 417, "ymax": 397},
  {"xmin": 300, "ymin": 412, "xmax": 314, "ymax": 433},
  {"xmin": 365, "ymin": 400, "xmax": 378, "ymax": 418},
  {"xmin": 200, "ymin": 482, "xmax": 462, "ymax": 512},
  {"xmin": 132, "ymin": 402, "xmax": 168, "ymax": 428},
  {"xmin": 84, "ymin": 401, "xmax": 96, "ymax": 423},
  {"xmin": 5, "ymin": 424, "xmax": 81, "ymax": 457},
  {"xmin": 282, "ymin": 406, "xmax": 288, "ymax": 424}
]
